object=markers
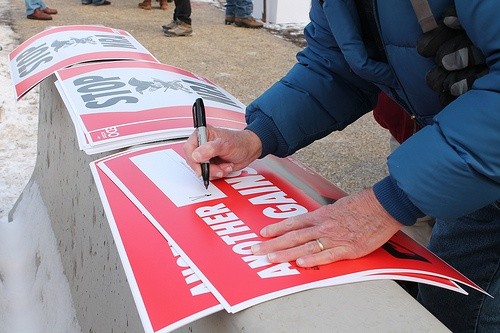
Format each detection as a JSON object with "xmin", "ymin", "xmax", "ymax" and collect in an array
[{"xmin": 192, "ymin": 98, "xmax": 211, "ymax": 191}]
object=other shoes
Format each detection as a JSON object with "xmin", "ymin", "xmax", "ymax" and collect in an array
[
  {"xmin": 162, "ymin": 20, "xmax": 192, "ymax": 37},
  {"xmin": 139, "ymin": 0, "xmax": 168, "ymax": 10},
  {"xmin": 82, "ymin": 0, "xmax": 111, "ymax": 6}
]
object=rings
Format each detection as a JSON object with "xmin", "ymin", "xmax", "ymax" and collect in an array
[{"xmin": 317, "ymin": 239, "xmax": 324, "ymax": 251}]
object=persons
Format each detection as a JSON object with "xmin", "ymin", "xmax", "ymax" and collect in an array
[
  {"xmin": 24, "ymin": 0, "xmax": 264, "ymax": 38},
  {"xmin": 181, "ymin": 0, "xmax": 500, "ymax": 333}
]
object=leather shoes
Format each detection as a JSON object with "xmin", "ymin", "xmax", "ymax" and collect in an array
[
  {"xmin": 224, "ymin": 16, "xmax": 263, "ymax": 28},
  {"xmin": 27, "ymin": 7, "xmax": 57, "ymax": 20}
]
